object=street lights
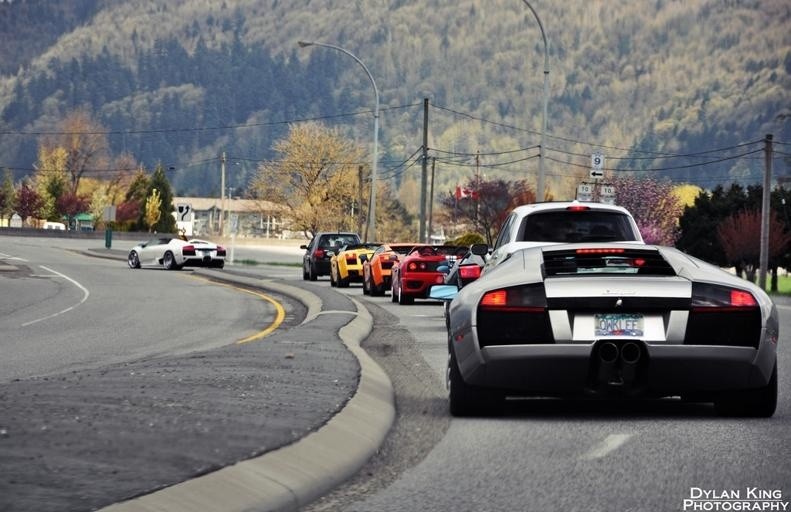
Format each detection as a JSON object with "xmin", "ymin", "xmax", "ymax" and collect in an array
[{"xmin": 299, "ymin": 38, "xmax": 380, "ymax": 241}]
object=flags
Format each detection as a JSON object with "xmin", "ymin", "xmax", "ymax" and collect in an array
[{"xmin": 455, "ymin": 186, "xmax": 480, "ymax": 201}]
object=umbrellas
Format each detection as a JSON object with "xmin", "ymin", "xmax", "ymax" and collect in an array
[{"xmin": 60, "ymin": 212, "xmax": 94, "ymax": 231}]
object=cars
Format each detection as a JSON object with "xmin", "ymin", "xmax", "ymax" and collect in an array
[{"xmin": 301, "ymin": 232, "xmax": 361, "ymax": 280}]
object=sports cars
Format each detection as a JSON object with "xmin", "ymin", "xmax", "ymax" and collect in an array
[{"xmin": 127, "ymin": 237, "xmax": 227, "ymax": 270}]
